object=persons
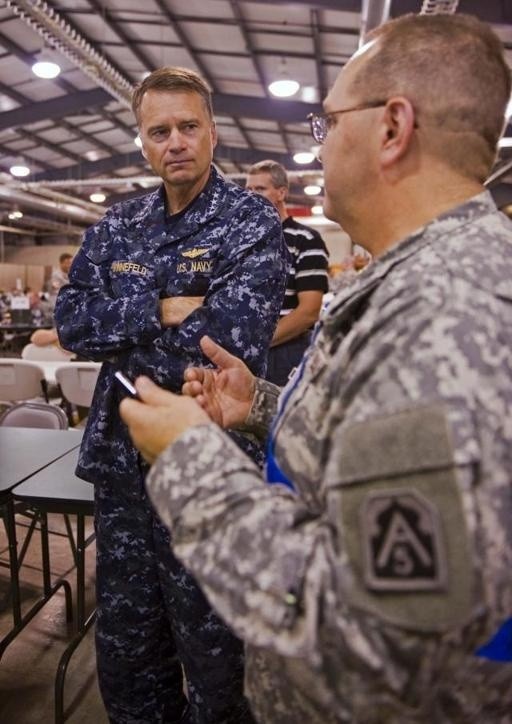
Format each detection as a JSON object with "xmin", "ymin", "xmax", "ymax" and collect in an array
[
  {"xmin": 51, "ymin": 64, "xmax": 296, "ymax": 724},
  {"xmin": 0, "ymin": 252, "xmax": 93, "ymax": 427},
  {"xmin": 241, "ymin": 159, "xmax": 332, "ymax": 386},
  {"xmin": 116, "ymin": 2, "xmax": 512, "ymax": 724},
  {"xmin": 324, "ymin": 243, "xmax": 371, "ymax": 302}
]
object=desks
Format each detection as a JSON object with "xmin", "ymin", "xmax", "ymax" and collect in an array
[{"xmin": 0, "ymin": 425, "xmax": 98, "ymax": 724}]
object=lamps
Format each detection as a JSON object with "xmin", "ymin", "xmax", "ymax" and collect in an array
[
  {"xmin": 89, "ymin": 186, "xmax": 106, "ymax": 204},
  {"xmin": 310, "ymin": 200, "xmax": 324, "ymax": 215},
  {"xmin": 7, "ymin": 203, "xmax": 24, "ymax": 220},
  {"xmin": 268, "ymin": 60, "xmax": 301, "ymax": 98},
  {"xmin": 290, "ymin": 140, "xmax": 314, "ymax": 166},
  {"xmin": 8, "ymin": 156, "xmax": 31, "ymax": 179},
  {"xmin": 31, "ymin": 48, "xmax": 62, "ymax": 78},
  {"xmin": 303, "ymin": 176, "xmax": 322, "ymax": 196}
]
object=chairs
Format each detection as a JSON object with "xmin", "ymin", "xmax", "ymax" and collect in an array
[{"xmin": 0, "ymin": 343, "xmax": 102, "ymax": 574}]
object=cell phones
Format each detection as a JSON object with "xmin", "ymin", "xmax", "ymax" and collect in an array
[{"xmin": 114, "ymin": 371, "xmax": 182, "ymax": 401}]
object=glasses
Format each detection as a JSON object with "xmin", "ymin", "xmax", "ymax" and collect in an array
[{"xmin": 306, "ymin": 95, "xmax": 422, "ymax": 148}]
